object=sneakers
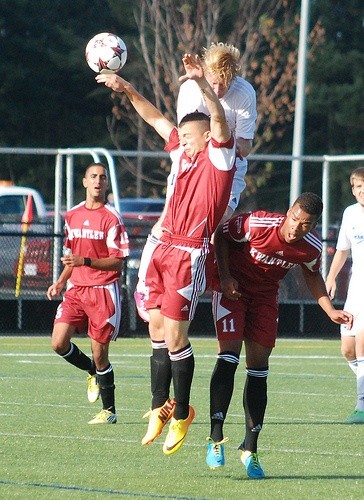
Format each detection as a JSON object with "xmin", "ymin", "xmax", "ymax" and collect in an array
[
  {"xmin": 88, "ymin": 405, "xmax": 117, "ymax": 424},
  {"xmin": 237, "ymin": 444, "xmax": 266, "ymax": 479},
  {"xmin": 86, "ymin": 372, "xmax": 100, "ymax": 403},
  {"xmin": 162, "ymin": 405, "xmax": 195, "ymax": 455},
  {"xmin": 345, "ymin": 411, "xmax": 364, "ymax": 424},
  {"xmin": 205, "ymin": 436, "xmax": 229, "ymax": 470},
  {"xmin": 141, "ymin": 398, "xmax": 176, "ymax": 446}
]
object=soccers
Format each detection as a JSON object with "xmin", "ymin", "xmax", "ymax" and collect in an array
[{"xmin": 85, "ymin": 33, "xmax": 127, "ymax": 74}]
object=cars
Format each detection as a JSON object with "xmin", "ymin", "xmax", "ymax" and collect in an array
[
  {"xmin": 1, "ymin": 181, "xmax": 166, "ymax": 288},
  {"xmin": 279, "ymin": 224, "xmax": 353, "ymax": 301}
]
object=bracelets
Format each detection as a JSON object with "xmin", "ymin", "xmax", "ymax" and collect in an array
[{"xmin": 84, "ymin": 257, "xmax": 91, "ymax": 266}]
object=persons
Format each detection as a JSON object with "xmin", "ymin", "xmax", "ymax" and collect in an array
[
  {"xmin": 45, "ymin": 163, "xmax": 131, "ymax": 424},
  {"xmin": 133, "ymin": 41, "xmax": 256, "ymax": 322},
  {"xmin": 96, "ymin": 53, "xmax": 237, "ymax": 454},
  {"xmin": 205, "ymin": 192, "xmax": 353, "ymax": 479},
  {"xmin": 322, "ymin": 166, "xmax": 364, "ymax": 425}
]
